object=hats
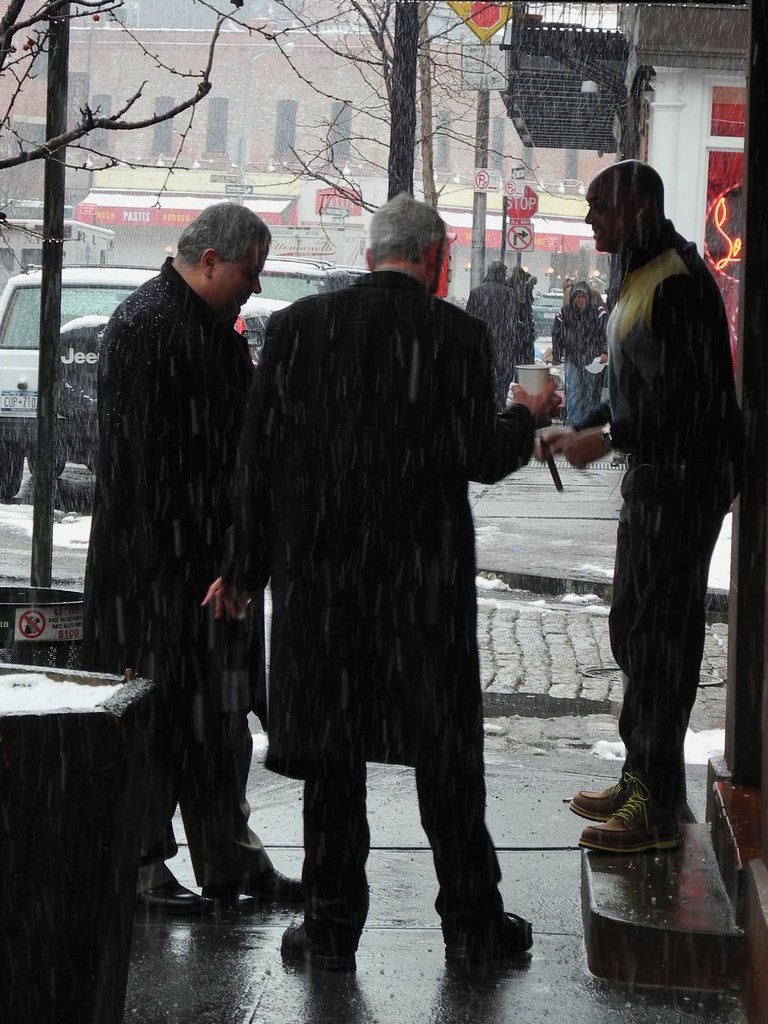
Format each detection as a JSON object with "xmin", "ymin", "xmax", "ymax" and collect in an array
[{"xmin": 488, "ymin": 261, "xmax": 507, "ymax": 273}]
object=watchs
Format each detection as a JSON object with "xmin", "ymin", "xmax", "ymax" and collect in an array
[{"xmin": 600, "ymin": 422, "xmax": 613, "ymax": 443}]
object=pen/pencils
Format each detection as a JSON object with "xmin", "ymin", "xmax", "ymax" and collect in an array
[{"xmin": 538, "ymin": 434, "xmax": 567, "ymax": 494}]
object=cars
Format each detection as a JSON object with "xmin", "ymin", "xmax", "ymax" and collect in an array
[
  {"xmin": 237, "ymin": 256, "xmax": 372, "ymax": 309},
  {"xmin": 532, "ymin": 287, "xmax": 564, "ymax": 333}
]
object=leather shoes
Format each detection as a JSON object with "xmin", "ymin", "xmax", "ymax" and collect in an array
[
  {"xmin": 202, "ymin": 869, "xmax": 304, "ymax": 902},
  {"xmin": 137, "ymin": 882, "xmax": 220, "ymax": 912}
]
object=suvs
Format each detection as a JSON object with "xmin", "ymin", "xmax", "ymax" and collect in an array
[{"xmin": 0, "ymin": 263, "xmax": 263, "ymax": 502}]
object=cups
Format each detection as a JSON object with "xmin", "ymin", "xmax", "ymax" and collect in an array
[{"xmin": 515, "ymin": 364, "xmax": 550, "ymax": 404}]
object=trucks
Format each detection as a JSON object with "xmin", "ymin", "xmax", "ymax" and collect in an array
[{"xmin": 0, "ymin": 219, "xmax": 116, "ymax": 294}]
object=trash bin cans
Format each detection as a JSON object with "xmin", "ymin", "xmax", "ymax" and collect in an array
[{"xmin": 0, "ymin": 585, "xmax": 84, "ymax": 669}]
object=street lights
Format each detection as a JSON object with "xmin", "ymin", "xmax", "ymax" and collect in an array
[{"xmin": 233, "ymin": 29, "xmax": 352, "ymax": 206}]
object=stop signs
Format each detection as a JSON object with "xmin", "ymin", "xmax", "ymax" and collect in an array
[{"xmin": 505, "ymin": 186, "xmax": 539, "ymax": 218}]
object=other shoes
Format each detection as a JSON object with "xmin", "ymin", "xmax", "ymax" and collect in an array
[
  {"xmin": 578, "ymin": 797, "xmax": 680, "ymax": 853},
  {"xmin": 569, "ymin": 780, "xmax": 633, "ymax": 823},
  {"xmin": 282, "ymin": 923, "xmax": 357, "ymax": 969},
  {"xmin": 447, "ymin": 915, "xmax": 534, "ymax": 962}
]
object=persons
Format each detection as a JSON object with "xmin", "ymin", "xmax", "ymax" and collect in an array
[
  {"xmin": 78, "ymin": 202, "xmax": 304, "ymax": 910},
  {"xmin": 550, "ymin": 282, "xmax": 611, "ymax": 424},
  {"xmin": 197, "ymin": 193, "xmax": 565, "ymax": 964},
  {"xmin": 530, "ymin": 162, "xmax": 743, "ymax": 854},
  {"xmin": 463, "ymin": 260, "xmax": 519, "ymax": 411},
  {"xmin": 509, "ymin": 265, "xmax": 607, "ymax": 365}
]
